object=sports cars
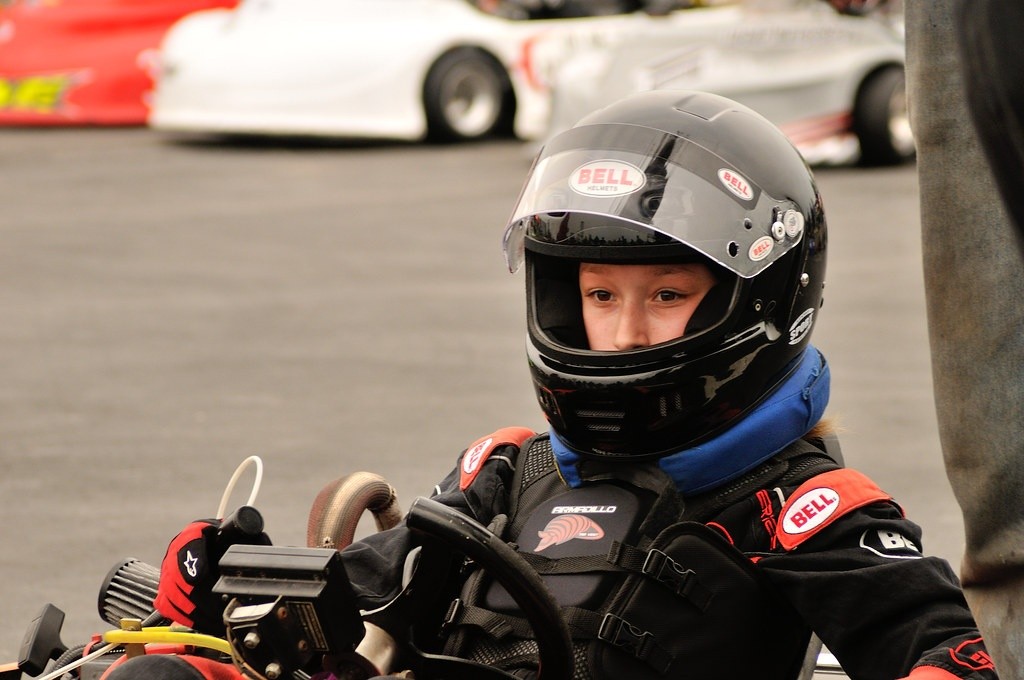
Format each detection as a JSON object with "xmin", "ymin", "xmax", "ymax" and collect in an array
[
  {"xmin": 138, "ymin": 1, "xmax": 921, "ymax": 168},
  {"xmin": 0, "ymin": 0, "xmax": 239, "ymax": 125}
]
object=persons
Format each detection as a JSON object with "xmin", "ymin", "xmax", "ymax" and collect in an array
[{"xmin": 108, "ymin": 87, "xmax": 1002, "ymax": 680}]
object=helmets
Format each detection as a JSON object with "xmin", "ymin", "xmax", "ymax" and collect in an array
[{"xmin": 523, "ymin": 90, "xmax": 827, "ymax": 459}]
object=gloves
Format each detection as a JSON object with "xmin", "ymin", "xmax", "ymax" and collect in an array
[{"xmin": 156, "ymin": 518, "xmax": 275, "ymax": 635}]
